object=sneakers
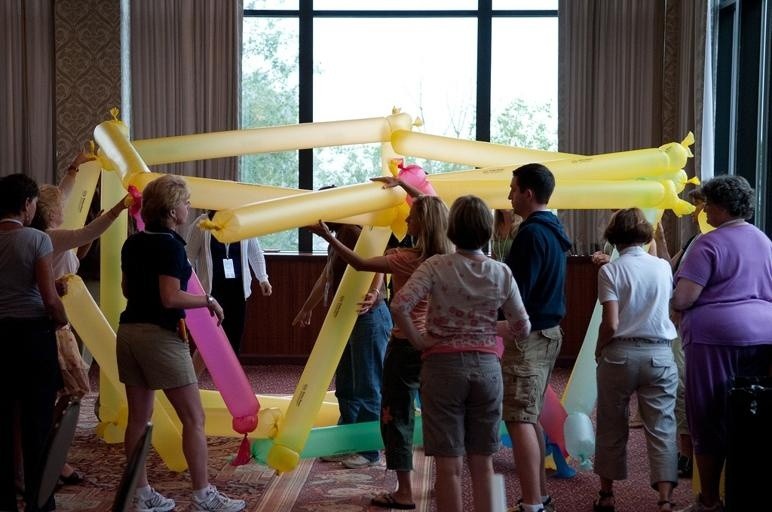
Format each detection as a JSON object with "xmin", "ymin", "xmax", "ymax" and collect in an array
[
  {"xmin": 189, "ymin": 485, "xmax": 245, "ymax": 512},
  {"xmin": 594, "ymin": 490, "xmax": 615, "ymax": 512},
  {"xmin": 133, "ymin": 488, "xmax": 175, "ymax": 512},
  {"xmin": 658, "ymin": 495, "xmax": 722, "ymax": 512},
  {"xmin": 320, "ymin": 452, "xmax": 381, "ymax": 468},
  {"xmin": 507, "ymin": 496, "xmax": 555, "ymax": 512},
  {"xmin": 677, "ymin": 453, "xmax": 691, "ymax": 477}
]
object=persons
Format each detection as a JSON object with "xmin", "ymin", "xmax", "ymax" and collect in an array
[
  {"xmin": 648, "ymin": 175, "xmax": 772, "ymax": 511},
  {"xmin": 186, "ymin": 208, "xmax": 271, "ymax": 377},
  {"xmin": 391, "ymin": 196, "xmax": 531, "ymax": 512},
  {"xmin": 1, "ymin": 139, "xmax": 129, "ymax": 511},
  {"xmin": 592, "ymin": 207, "xmax": 690, "ymax": 510},
  {"xmin": 493, "ymin": 162, "xmax": 571, "ymax": 511},
  {"xmin": 117, "ymin": 176, "xmax": 246, "ymax": 512},
  {"xmin": 308, "ymin": 176, "xmax": 457, "ymax": 509},
  {"xmin": 293, "ymin": 183, "xmax": 392, "ymax": 468}
]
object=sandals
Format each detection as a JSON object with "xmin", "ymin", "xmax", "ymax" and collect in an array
[{"xmin": 62, "ymin": 470, "xmax": 84, "ymax": 482}]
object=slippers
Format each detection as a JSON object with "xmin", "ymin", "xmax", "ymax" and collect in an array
[{"xmin": 372, "ymin": 494, "xmax": 415, "ymax": 510}]
